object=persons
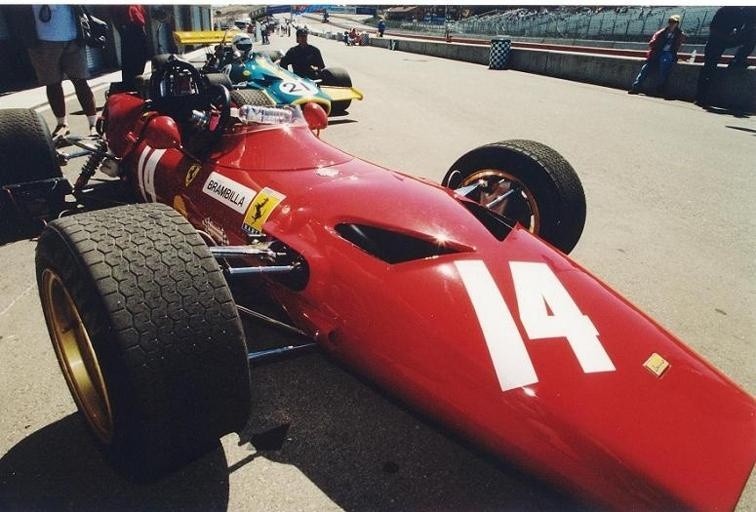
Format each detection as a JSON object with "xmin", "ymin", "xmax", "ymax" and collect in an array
[
  {"xmin": 693, "ymin": 7, "xmax": 756, "ymax": 107},
  {"xmin": 627, "ymin": 15, "xmax": 689, "ymax": 98},
  {"xmin": 216, "ymin": 7, "xmax": 386, "ymax": 45},
  {"xmin": 411, "ymin": 6, "xmax": 653, "ymax": 43},
  {"xmin": 230, "ymin": 35, "xmax": 254, "ymax": 61},
  {"xmin": 10, "ymin": 5, "xmax": 101, "ymax": 142},
  {"xmin": 111, "ymin": 5, "xmax": 174, "ymax": 84},
  {"xmin": 280, "ymin": 26, "xmax": 325, "ymax": 80}
]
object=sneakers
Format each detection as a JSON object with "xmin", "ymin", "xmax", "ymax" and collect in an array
[
  {"xmin": 694, "ymin": 99, "xmax": 716, "ymax": 112},
  {"xmin": 628, "ymin": 88, "xmax": 639, "ymax": 95},
  {"xmin": 51, "ymin": 124, "xmax": 72, "ymax": 140},
  {"xmin": 88, "ymin": 123, "xmax": 102, "ymax": 140}
]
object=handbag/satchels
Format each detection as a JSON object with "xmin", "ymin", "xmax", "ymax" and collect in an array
[{"xmin": 77, "ymin": 11, "xmax": 109, "ymax": 50}]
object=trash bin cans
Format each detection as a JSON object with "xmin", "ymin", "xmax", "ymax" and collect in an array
[
  {"xmin": 361, "ymin": 33, "xmax": 369, "ymax": 46},
  {"xmin": 489, "ymin": 38, "xmax": 511, "ymax": 70}
]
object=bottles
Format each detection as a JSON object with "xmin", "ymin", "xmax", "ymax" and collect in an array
[{"xmin": 229, "ymin": 105, "xmax": 298, "ymax": 126}]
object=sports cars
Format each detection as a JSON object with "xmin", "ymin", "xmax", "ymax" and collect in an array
[{"xmin": 0, "ymin": 48, "xmax": 756, "ymax": 511}]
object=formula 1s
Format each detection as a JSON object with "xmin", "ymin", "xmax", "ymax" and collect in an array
[{"xmin": 168, "ymin": 27, "xmax": 364, "ymax": 120}]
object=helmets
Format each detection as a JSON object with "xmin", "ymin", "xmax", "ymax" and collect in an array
[
  {"xmin": 230, "ymin": 32, "xmax": 253, "ymax": 56},
  {"xmin": 296, "ymin": 28, "xmax": 308, "ymax": 36}
]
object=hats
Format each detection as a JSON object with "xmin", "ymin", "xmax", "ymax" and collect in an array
[{"xmin": 669, "ymin": 15, "xmax": 681, "ymax": 23}]
